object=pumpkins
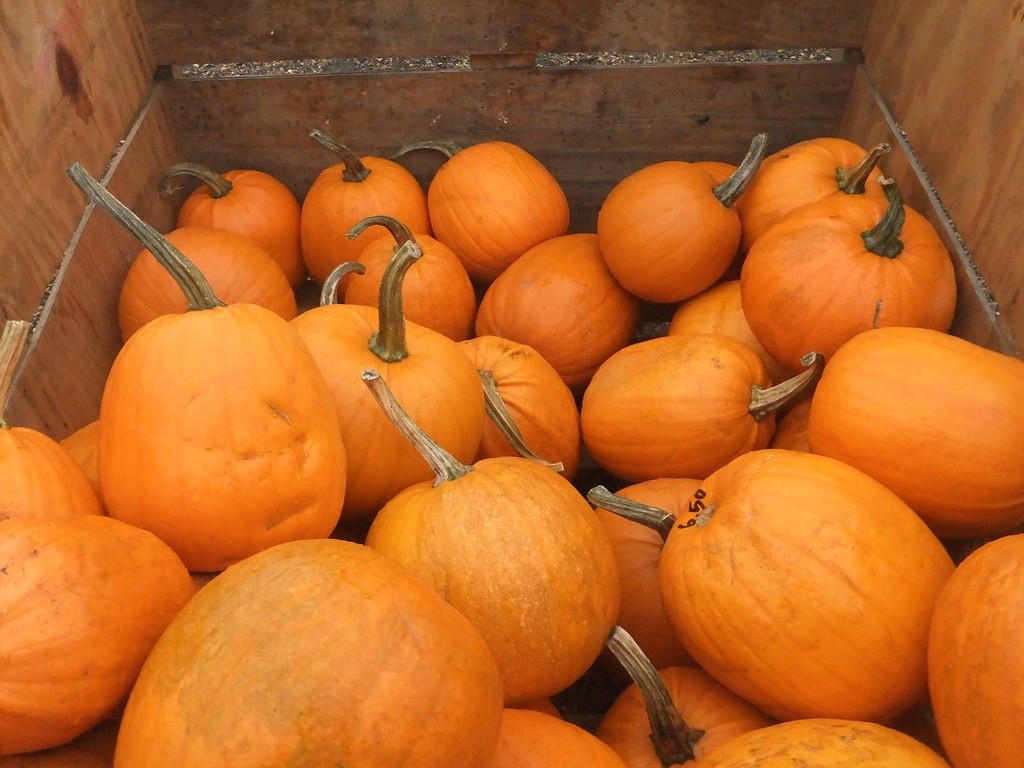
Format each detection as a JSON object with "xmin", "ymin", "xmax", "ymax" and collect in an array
[{"xmin": 0, "ymin": 131, "xmax": 1024, "ymax": 767}]
